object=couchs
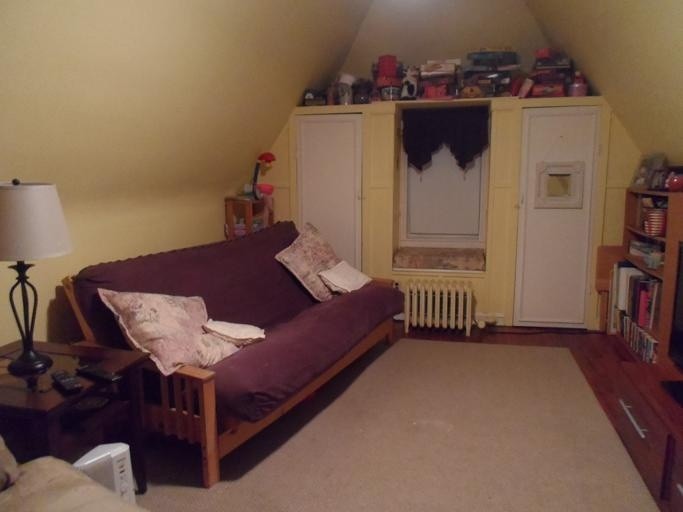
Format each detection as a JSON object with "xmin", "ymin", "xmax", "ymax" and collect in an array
[{"xmin": 58, "ymin": 221, "xmax": 401, "ymax": 488}]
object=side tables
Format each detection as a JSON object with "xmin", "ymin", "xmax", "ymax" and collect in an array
[{"xmin": 2, "ymin": 340, "xmax": 151, "ymax": 501}]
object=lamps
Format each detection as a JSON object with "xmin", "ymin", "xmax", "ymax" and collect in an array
[{"xmin": 2, "ymin": 179, "xmax": 74, "ymax": 392}]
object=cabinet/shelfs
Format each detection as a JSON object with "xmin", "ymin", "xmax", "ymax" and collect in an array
[
  {"xmin": 568, "ymin": 334, "xmax": 683, "ymax": 511},
  {"xmin": 606, "ymin": 180, "xmax": 682, "ymax": 364}
]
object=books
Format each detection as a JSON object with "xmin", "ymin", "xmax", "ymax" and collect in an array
[{"xmin": 609, "ymin": 258, "xmax": 660, "ymax": 365}]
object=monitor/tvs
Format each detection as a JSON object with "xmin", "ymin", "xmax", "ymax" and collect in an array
[{"xmin": 659, "ymin": 240, "xmax": 683, "ymax": 408}]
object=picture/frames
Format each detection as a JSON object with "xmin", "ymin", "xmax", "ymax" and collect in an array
[{"xmin": 533, "ymin": 160, "xmax": 586, "ymax": 210}]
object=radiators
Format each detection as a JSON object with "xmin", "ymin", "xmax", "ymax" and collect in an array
[{"xmin": 401, "ymin": 279, "xmax": 475, "ymax": 338}]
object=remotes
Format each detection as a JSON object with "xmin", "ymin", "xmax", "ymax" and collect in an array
[
  {"xmin": 50, "ymin": 367, "xmax": 84, "ymax": 395},
  {"xmin": 75, "ymin": 361, "xmax": 125, "ymax": 383}
]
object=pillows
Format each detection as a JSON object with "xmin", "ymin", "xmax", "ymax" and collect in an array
[
  {"xmin": 275, "ymin": 222, "xmax": 370, "ymax": 303},
  {"xmin": 94, "ymin": 283, "xmax": 264, "ymax": 376}
]
